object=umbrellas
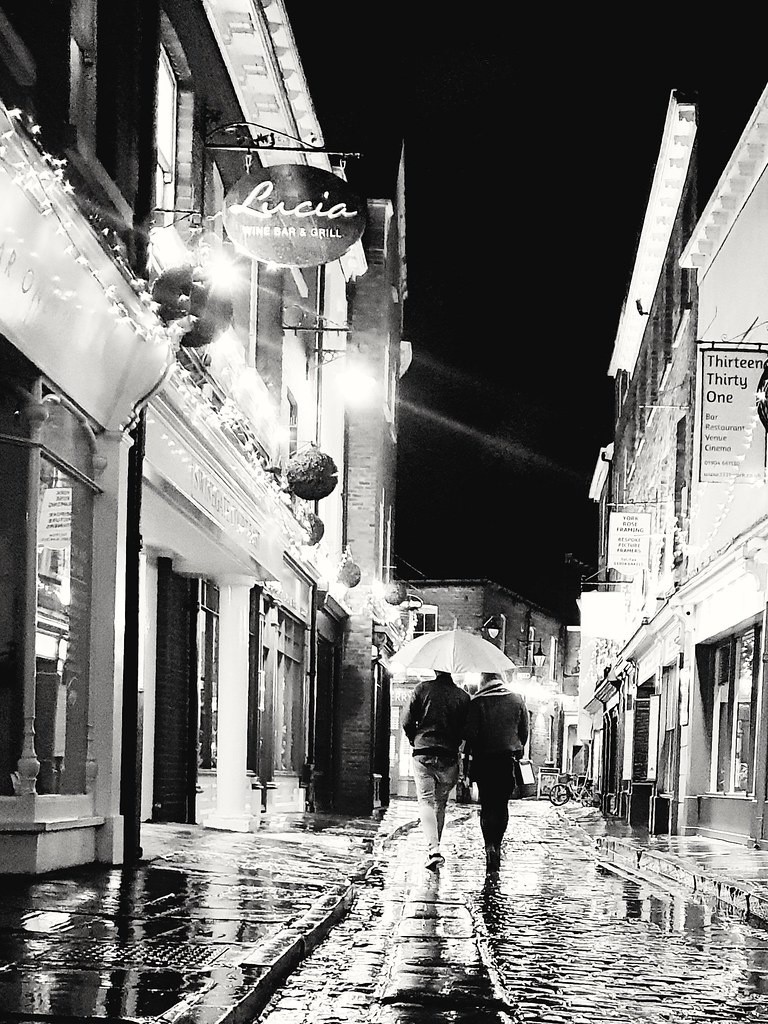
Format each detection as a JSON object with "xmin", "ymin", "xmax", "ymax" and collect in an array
[{"xmin": 387, "ymin": 614, "xmax": 518, "ymax": 675}]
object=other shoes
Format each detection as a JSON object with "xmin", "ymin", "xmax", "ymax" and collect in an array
[{"xmin": 486, "ymin": 846, "xmax": 496, "ymax": 873}]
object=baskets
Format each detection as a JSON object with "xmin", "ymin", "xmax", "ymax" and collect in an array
[{"xmin": 558, "ymin": 774, "xmax": 569, "ymax": 782}]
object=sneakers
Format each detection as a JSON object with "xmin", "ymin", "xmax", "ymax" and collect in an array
[{"xmin": 425, "ymin": 853, "xmax": 444, "ymax": 872}]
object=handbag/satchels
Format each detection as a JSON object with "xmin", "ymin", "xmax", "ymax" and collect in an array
[{"xmin": 512, "ymin": 758, "xmax": 537, "ymax": 787}]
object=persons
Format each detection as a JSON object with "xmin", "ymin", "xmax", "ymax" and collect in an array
[
  {"xmin": 402, "ymin": 671, "xmax": 470, "ymax": 868},
  {"xmin": 464, "ymin": 672, "xmax": 528, "ymax": 872}
]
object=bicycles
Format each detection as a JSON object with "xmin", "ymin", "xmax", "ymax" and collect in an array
[{"xmin": 549, "ymin": 775, "xmax": 599, "ymax": 807}]
